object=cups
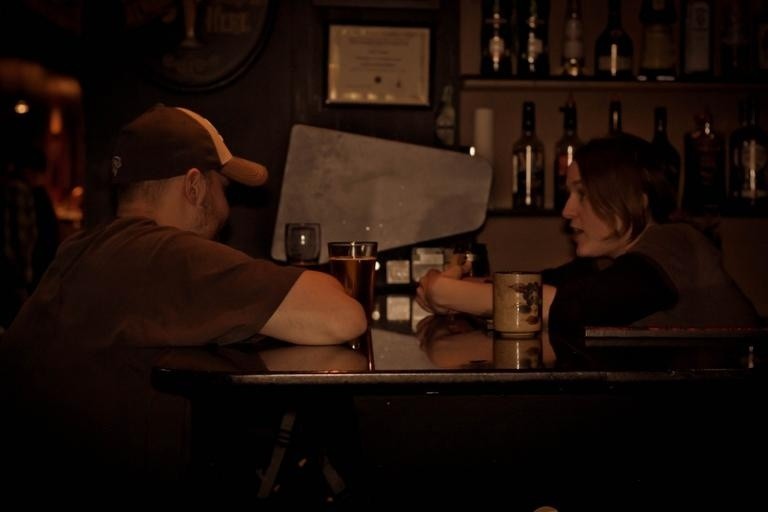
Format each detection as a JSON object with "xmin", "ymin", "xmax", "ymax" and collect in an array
[
  {"xmin": 284, "ymin": 221, "xmax": 321, "ymax": 267},
  {"xmin": 327, "ymin": 241, "xmax": 377, "ymax": 337},
  {"xmin": 492, "ymin": 271, "xmax": 543, "ymax": 340}
]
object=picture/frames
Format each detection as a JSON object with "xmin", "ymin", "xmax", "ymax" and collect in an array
[{"xmin": 320, "ymin": 18, "xmax": 437, "ymax": 112}]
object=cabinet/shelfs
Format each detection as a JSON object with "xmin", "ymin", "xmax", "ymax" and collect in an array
[{"xmin": 460, "ymin": 74, "xmax": 767, "ymax": 225}]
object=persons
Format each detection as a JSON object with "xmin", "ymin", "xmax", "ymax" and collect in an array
[
  {"xmin": 415, "ymin": 313, "xmax": 767, "ymax": 369},
  {"xmin": 0, "ymin": 98, "xmax": 371, "ymax": 509},
  {"xmin": 413, "ymin": 135, "xmax": 762, "ymax": 333}
]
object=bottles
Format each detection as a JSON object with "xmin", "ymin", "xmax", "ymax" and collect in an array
[
  {"xmin": 553, "ymin": 100, "xmax": 582, "ymax": 209},
  {"xmin": 477, "ymin": 0, "xmax": 761, "ymax": 80},
  {"xmin": 606, "ymin": 100, "xmax": 768, "ymax": 209},
  {"xmin": 512, "ymin": 100, "xmax": 546, "ymax": 208}
]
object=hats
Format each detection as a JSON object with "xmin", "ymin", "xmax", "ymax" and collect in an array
[{"xmin": 109, "ymin": 102, "xmax": 269, "ymax": 186}]
